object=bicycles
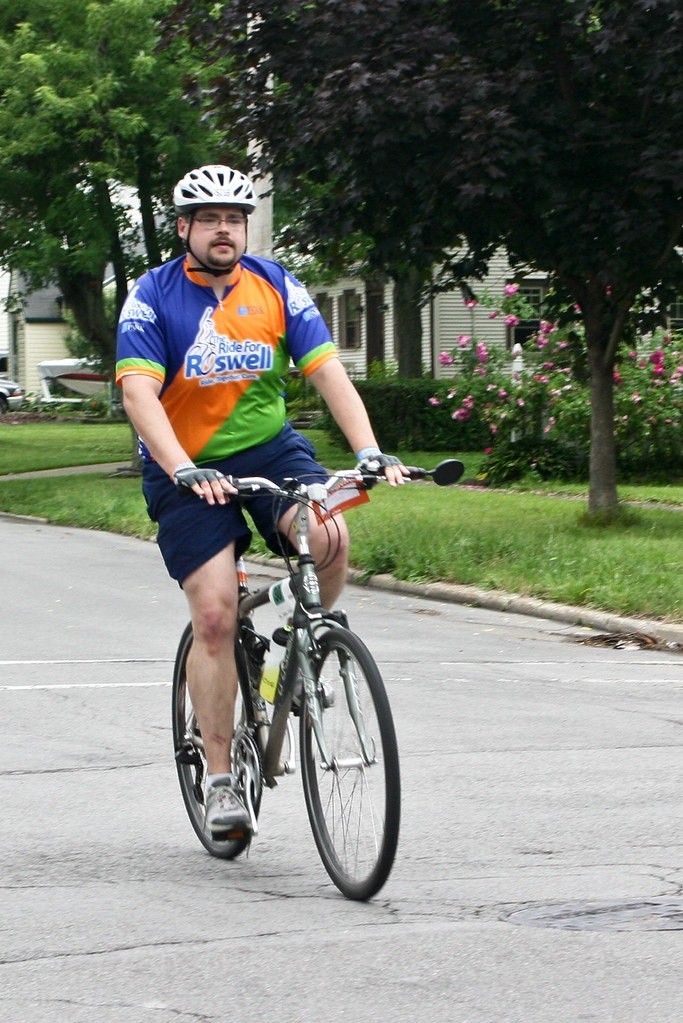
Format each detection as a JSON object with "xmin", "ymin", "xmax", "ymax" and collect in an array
[{"xmin": 166, "ymin": 455, "xmax": 468, "ymax": 903}]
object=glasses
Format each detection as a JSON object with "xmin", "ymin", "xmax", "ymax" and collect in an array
[{"xmin": 188, "ymin": 211, "xmax": 247, "ymax": 230}]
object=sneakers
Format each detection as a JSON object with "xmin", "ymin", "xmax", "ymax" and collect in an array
[
  {"xmin": 202, "ymin": 776, "xmax": 251, "ymax": 833},
  {"xmin": 279, "ymin": 661, "xmax": 336, "ymax": 709}
]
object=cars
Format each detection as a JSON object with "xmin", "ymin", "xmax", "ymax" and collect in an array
[{"xmin": 1, "ymin": 375, "xmax": 23, "ymax": 416}]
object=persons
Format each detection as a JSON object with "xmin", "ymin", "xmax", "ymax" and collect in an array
[{"xmin": 115, "ymin": 164, "xmax": 408, "ymax": 837}]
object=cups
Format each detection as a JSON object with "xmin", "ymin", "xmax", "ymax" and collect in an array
[{"xmin": 259, "ymin": 622, "xmax": 293, "ymax": 706}]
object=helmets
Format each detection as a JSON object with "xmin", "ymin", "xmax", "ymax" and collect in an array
[{"xmin": 173, "ymin": 163, "xmax": 257, "ymax": 218}]
